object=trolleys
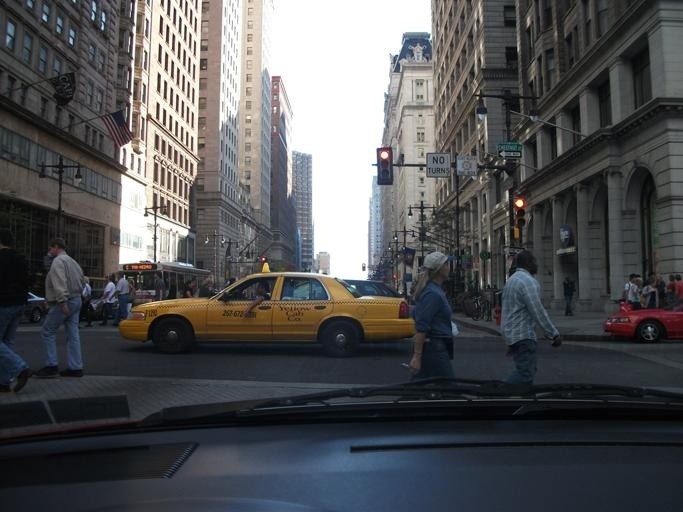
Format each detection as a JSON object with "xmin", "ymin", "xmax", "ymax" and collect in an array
[{"xmin": 79, "ymin": 296, "xmax": 109, "ymax": 323}]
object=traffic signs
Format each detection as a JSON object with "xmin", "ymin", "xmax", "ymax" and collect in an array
[
  {"xmin": 425, "ymin": 152, "xmax": 450, "ymax": 179},
  {"xmin": 499, "ymin": 151, "xmax": 522, "ymax": 158},
  {"xmin": 455, "ymin": 155, "xmax": 478, "ymax": 176},
  {"xmin": 496, "ymin": 143, "xmax": 523, "ymax": 151}
]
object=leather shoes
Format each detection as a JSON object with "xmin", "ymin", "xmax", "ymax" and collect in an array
[{"xmin": 0, "ymin": 365, "xmax": 82, "ymax": 394}]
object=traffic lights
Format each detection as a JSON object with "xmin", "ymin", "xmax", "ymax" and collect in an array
[
  {"xmin": 361, "ymin": 262, "xmax": 366, "ymax": 271},
  {"xmin": 514, "ymin": 195, "xmax": 529, "ymax": 228},
  {"xmin": 376, "ymin": 146, "xmax": 393, "ymax": 186}
]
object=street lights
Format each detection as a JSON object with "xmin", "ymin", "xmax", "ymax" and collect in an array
[
  {"xmin": 404, "ymin": 198, "xmax": 439, "ymax": 256},
  {"xmin": 391, "ymin": 225, "xmax": 416, "ymax": 286},
  {"xmin": 220, "ymin": 236, "xmax": 260, "ymax": 282},
  {"xmin": 367, "ymin": 235, "xmax": 400, "ymax": 293},
  {"xmin": 203, "ymin": 229, "xmax": 227, "ymax": 280},
  {"xmin": 36, "ymin": 150, "xmax": 88, "ymax": 238},
  {"xmin": 472, "ymin": 88, "xmax": 544, "ymax": 258},
  {"xmin": 143, "ymin": 203, "xmax": 170, "ymax": 263}
]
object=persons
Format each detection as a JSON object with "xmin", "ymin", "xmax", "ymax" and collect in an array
[
  {"xmin": 153, "ymin": 270, "xmax": 166, "ymax": 300},
  {"xmin": 182, "ymin": 279, "xmax": 195, "ymax": 298},
  {"xmin": 623, "ymin": 271, "xmax": 683, "ymax": 309},
  {"xmin": 225, "ymin": 277, "xmax": 243, "ymax": 299},
  {"xmin": 79, "ymin": 276, "xmax": 94, "ymax": 329},
  {"xmin": 563, "ymin": 276, "xmax": 575, "ymax": 316},
  {"xmin": 0, "ymin": 228, "xmax": 32, "ymax": 393},
  {"xmin": 242, "ymin": 280, "xmax": 272, "ymax": 319},
  {"xmin": 99, "ymin": 270, "xmax": 136, "ymax": 327},
  {"xmin": 198, "ymin": 278, "xmax": 215, "ymax": 297},
  {"xmin": 501, "ymin": 249, "xmax": 561, "ymax": 385},
  {"xmin": 167, "ymin": 275, "xmax": 177, "ymax": 299},
  {"xmin": 32, "ymin": 237, "xmax": 86, "ymax": 378},
  {"xmin": 401, "ymin": 252, "xmax": 454, "ymax": 382}
]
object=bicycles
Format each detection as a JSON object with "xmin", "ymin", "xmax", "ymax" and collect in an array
[{"xmin": 462, "ymin": 286, "xmax": 495, "ymax": 322}]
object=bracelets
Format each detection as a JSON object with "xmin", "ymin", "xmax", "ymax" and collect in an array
[{"xmin": 413, "ymin": 349, "xmax": 423, "ymax": 356}]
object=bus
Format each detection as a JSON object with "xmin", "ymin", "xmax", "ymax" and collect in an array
[{"xmin": 121, "ymin": 260, "xmax": 214, "ymax": 311}]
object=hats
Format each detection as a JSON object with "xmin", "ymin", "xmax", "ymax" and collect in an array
[{"xmin": 424, "ymin": 252, "xmax": 448, "ymax": 274}]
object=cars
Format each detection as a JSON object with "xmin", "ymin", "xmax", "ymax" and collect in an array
[
  {"xmin": 18, "ymin": 291, "xmax": 48, "ymax": 323},
  {"xmin": 117, "ymin": 259, "xmax": 416, "ymax": 359},
  {"xmin": 329, "ymin": 273, "xmax": 402, "ymax": 298},
  {"xmin": 601, "ymin": 295, "xmax": 682, "ymax": 344}
]
